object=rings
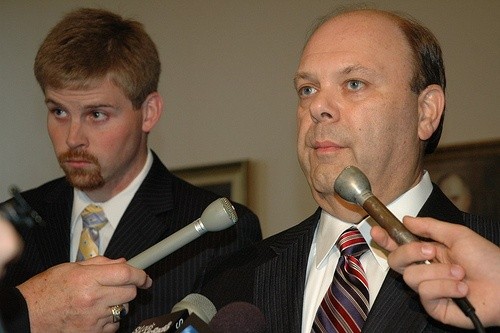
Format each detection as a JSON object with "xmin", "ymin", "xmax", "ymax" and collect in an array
[{"xmin": 112, "ymin": 304, "xmax": 126, "ymax": 322}]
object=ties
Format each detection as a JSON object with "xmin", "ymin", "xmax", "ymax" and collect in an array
[
  {"xmin": 310, "ymin": 229, "xmax": 369, "ymax": 333},
  {"xmin": 76, "ymin": 204, "xmax": 108, "ymax": 263}
]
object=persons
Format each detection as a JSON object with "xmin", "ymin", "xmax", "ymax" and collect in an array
[
  {"xmin": 245, "ymin": 7, "xmax": 500, "ymax": 333},
  {"xmin": 371, "ymin": 216, "xmax": 500, "ymax": 329},
  {"xmin": 0, "ymin": 255, "xmax": 152, "ymax": 333},
  {"xmin": 0, "ymin": 8, "xmax": 262, "ymax": 333}
]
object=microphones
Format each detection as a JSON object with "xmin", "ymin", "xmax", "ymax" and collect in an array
[
  {"xmin": 334, "ymin": 165, "xmax": 473, "ymax": 315},
  {"xmin": 126, "ymin": 196, "xmax": 238, "ymax": 270},
  {"xmin": 132, "ymin": 292, "xmax": 216, "ymax": 333},
  {"xmin": 208, "ymin": 301, "xmax": 265, "ymax": 333}
]
